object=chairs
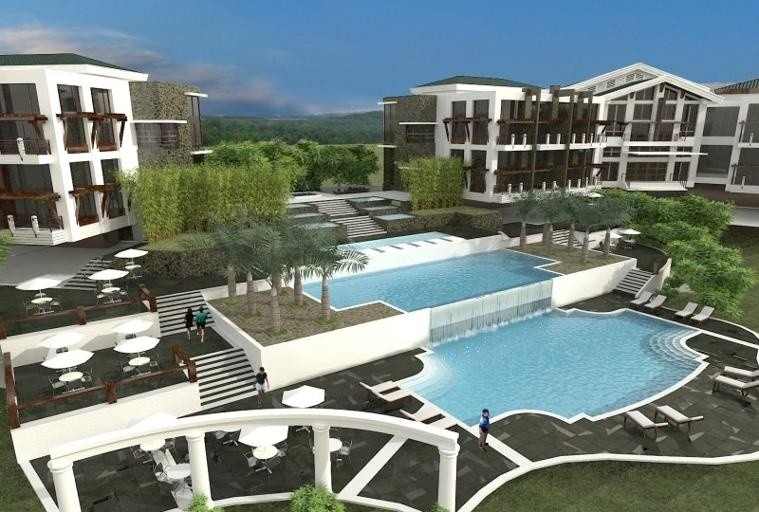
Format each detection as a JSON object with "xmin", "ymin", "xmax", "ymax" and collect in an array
[
  {"xmin": 622, "ymin": 291, "xmax": 759, "ymax": 441},
  {"xmin": 24, "ymin": 260, "xmax": 457, "ymax": 493}
]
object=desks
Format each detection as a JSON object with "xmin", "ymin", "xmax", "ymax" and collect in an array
[
  {"xmin": 625, "ymin": 240, "xmax": 634, "ymax": 249},
  {"xmin": 610, "ymin": 244, "xmax": 615, "ymax": 252}
]
